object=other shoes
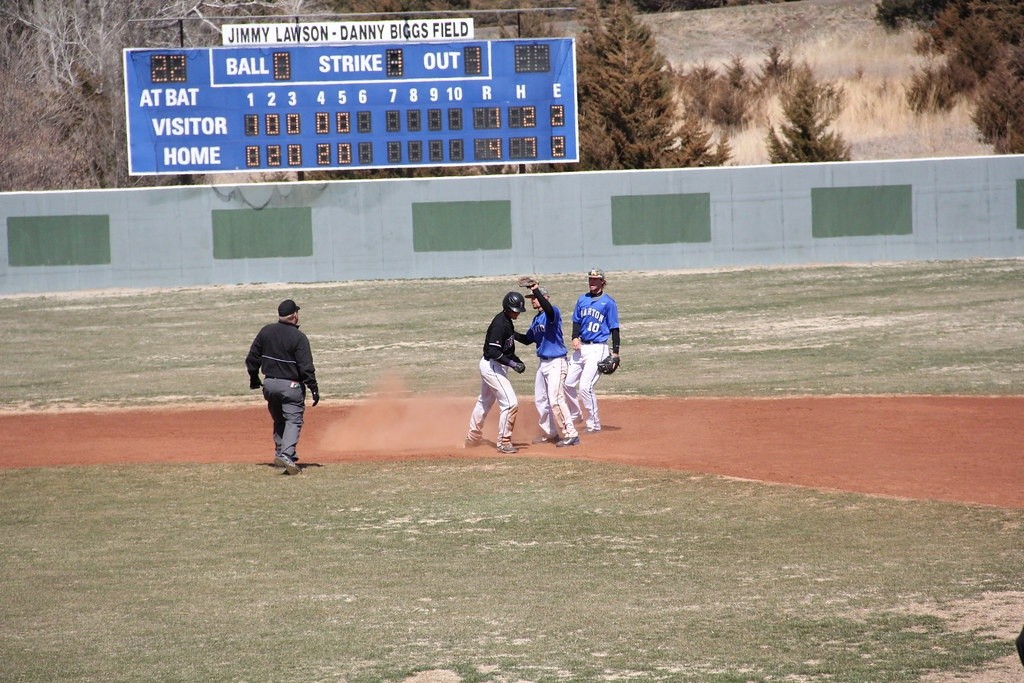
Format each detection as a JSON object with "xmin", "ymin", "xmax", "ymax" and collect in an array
[
  {"xmin": 572, "ymin": 415, "xmax": 584, "ymax": 424},
  {"xmin": 275, "ymin": 451, "xmax": 299, "ymax": 466},
  {"xmin": 583, "ymin": 425, "xmax": 600, "ymax": 433},
  {"xmin": 282, "ymin": 454, "xmax": 301, "ymax": 475}
]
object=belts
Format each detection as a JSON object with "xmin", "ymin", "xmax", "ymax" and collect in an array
[
  {"xmin": 266, "ymin": 375, "xmax": 292, "ymax": 380},
  {"xmin": 581, "ymin": 338, "xmax": 606, "ymax": 343},
  {"xmin": 542, "ymin": 357, "xmax": 557, "ymax": 360},
  {"xmin": 483, "ymin": 355, "xmax": 490, "ymax": 361}
]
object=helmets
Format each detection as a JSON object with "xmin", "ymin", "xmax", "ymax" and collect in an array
[{"xmin": 502, "ymin": 292, "xmax": 526, "ymax": 312}]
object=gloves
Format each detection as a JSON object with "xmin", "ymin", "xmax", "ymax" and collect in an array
[
  {"xmin": 312, "ymin": 391, "xmax": 319, "ymax": 406},
  {"xmin": 513, "ymin": 357, "xmax": 525, "ymax": 374},
  {"xmin": 250, "ymin": 375, "xmax": 263, "ymax": 389}
]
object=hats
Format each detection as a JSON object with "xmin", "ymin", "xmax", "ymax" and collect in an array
[
  {"xmin": 588, "ymin": 267, "xmax": 604, "ymax": 278},
  {"xmin": 278, "ymin": 300, "xmax": 300, "ymax": 316},
  {"xmin": 525, "ymin": 287, "xmax": 549, "ymax": 298}
]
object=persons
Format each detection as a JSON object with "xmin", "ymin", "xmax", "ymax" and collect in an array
[
  {"xmin": 464, "ymin": 292, "xmax": 526, "ymax": 453},
  {"xmin": 246, "ymin": 300, "xmax": 320, "ymax": 475},
  {"xmin": 513, "ymin": 276, "xmax": 580, "ymax": 448},
  {"xmin": 564, "ymin": 269, "xmax": 620, "ymax": 433}
]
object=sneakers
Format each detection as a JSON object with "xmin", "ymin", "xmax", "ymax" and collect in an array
[
  {"xmin": 497, "ymin": 445, "xmax": 518, "ymax": 453},
  {"xmin": 556, "ymin": 436, "xmax": 580, "ymax": 447},
  {"xmin": 465, "ymin": 437, "xmax": 490, "ymax": 448},
  {"xmin": 532, "ymin": 434, "xmax": 559, "ymax": 444}
]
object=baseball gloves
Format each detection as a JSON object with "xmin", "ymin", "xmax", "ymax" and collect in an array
[
  {"xmin": 597, "ymin": 355, "xmax": 621, "ymax": 375},
  {"xmin": 517, "ymin": 275, "xmax": 539, "ymax": 289}
]
